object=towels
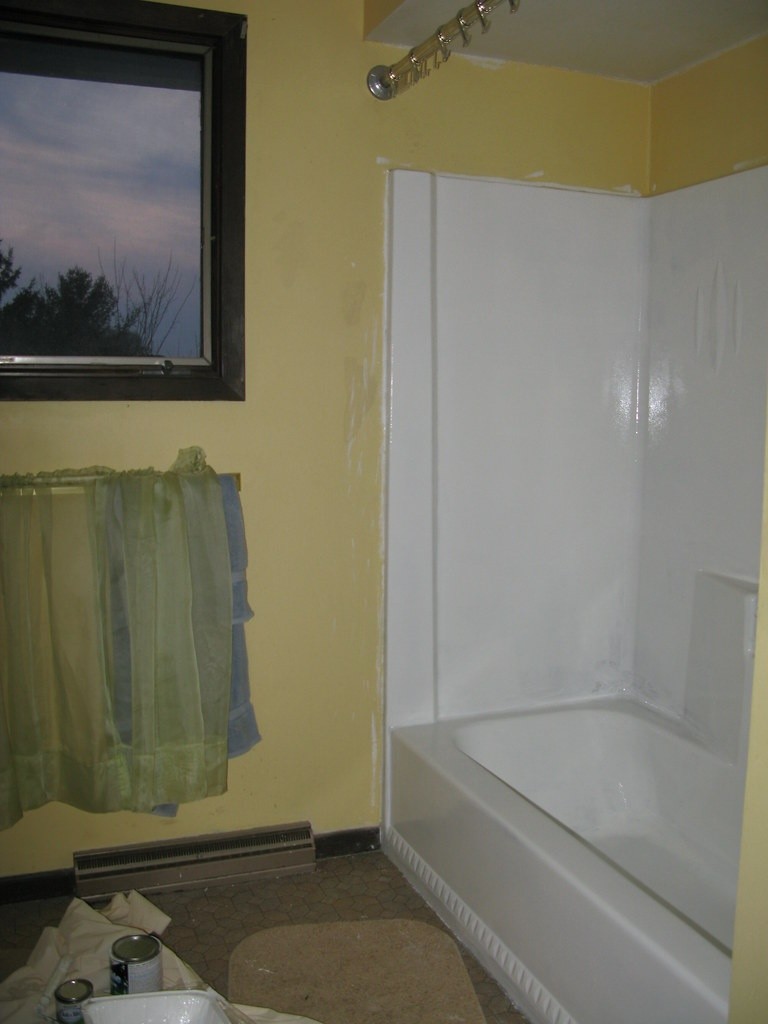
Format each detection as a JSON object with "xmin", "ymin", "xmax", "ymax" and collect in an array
[{"xmin": 0, "ymin": 445, "xmax": 261, "ymax": 833}]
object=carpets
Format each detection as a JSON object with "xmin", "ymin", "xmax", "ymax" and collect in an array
[{"xmin": 226, "ymin": 917, "xmax": 488, "ymax": 1024}]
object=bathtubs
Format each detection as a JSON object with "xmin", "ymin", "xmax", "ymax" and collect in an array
[{"xmin": 388, "ymin": 693, "xmax": 745, "ymax": 1024}]
